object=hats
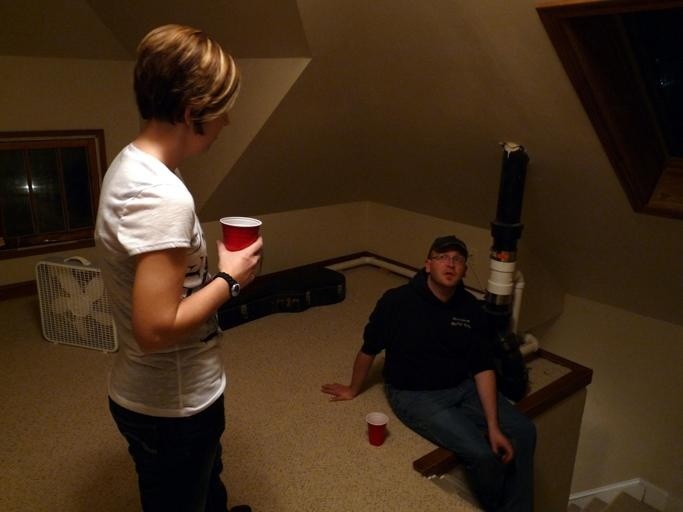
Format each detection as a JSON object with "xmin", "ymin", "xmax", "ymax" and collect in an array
[{"xmin": 428, "ymin": 236, "xmax": 467, "ymax": 259}]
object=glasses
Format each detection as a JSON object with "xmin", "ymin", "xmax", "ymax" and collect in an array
[{"xmin": 433, "ymin": 255, "xmax": 465, "ymax": 263}]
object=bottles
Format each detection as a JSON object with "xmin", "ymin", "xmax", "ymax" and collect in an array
[
  {"xmin": 365, "ymin": 411, "xmax": 389, "ymax": 446},
  {"xmin": 219, "ymin": 215, "xmax": 262, "ymax": 252}
]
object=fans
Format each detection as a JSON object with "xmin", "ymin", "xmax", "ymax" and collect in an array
[{"xmin": 35, "ymin": 253, "xmax": 119, "ymax": 354}]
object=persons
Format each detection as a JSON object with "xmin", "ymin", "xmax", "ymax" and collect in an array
[
  {"xmin": 93, "ymin": 25, "xmax": 263, "ymax": 511},
  {"xmin": 322, "ymin": 235, "xmax": 536, "ymax": 510}
]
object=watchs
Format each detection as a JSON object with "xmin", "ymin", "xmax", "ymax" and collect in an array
[{"xmin": 217, "ymin": 271, "xmax": 241, "ymax": 298}]
